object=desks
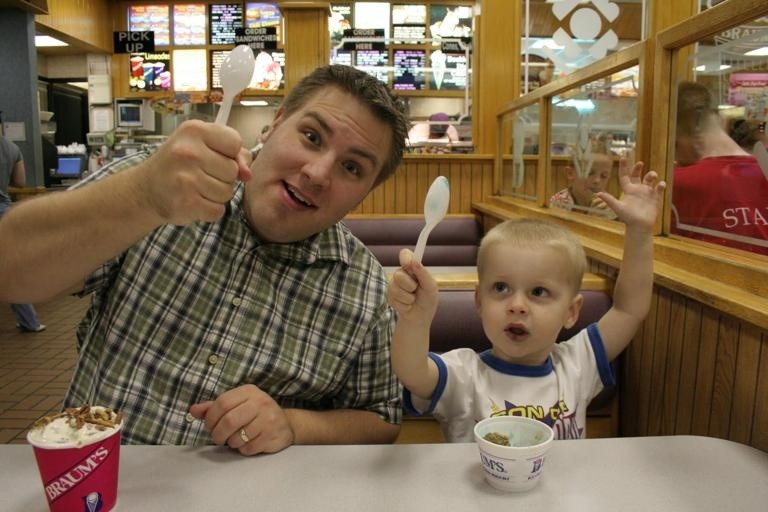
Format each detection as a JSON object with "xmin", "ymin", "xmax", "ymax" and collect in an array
[
  {"xmin": 374, "ymin": 264, "xmax": 609, "ymax": 295},
  {"xmin": 0, "ymin": 430, "xmax": 768, "ymax": 512}
]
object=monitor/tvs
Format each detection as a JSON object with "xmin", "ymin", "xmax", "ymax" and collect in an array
[
  {"xmin": 56, "ymin": 155, "xmax": 85, "ymax": 178},
  {"xmin": 117, "ymin": 103, "xmax": 143, "ymax": 127}
]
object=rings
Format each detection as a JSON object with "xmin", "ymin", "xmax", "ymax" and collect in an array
[{"xmin": 238, "ymin": 426, "xmax": 251, "ymax": 443}]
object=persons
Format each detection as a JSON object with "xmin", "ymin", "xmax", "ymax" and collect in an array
[
  {"xmin": 1, "ymin": 132, "xmax": 47, "ymax": 333},
  {"xmin": 1, "ymin": 62, "xmax": 405, "ymax": 457},
  {"xmin": 669, "ymin": 81, "xmax": 767, "ymax": 255},
  {"xmin": 384, "ymin": 155, "xmax": 667, "ymax": 441},
  {"xmin": 547, "ymin": 137, "xmax": 623, "ymax": 223},
  {"xmin": 408, "ymin": 112, "xmax": 461, "ymax": 142}
]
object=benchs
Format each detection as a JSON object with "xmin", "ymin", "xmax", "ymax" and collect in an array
[
  {"xmin": 371, "ymin": 288, "xmax": 620, "ymax": 442},
  {"xmin": 330, "ymin": 207, "xmax": 486, "ymax": 265}
]
